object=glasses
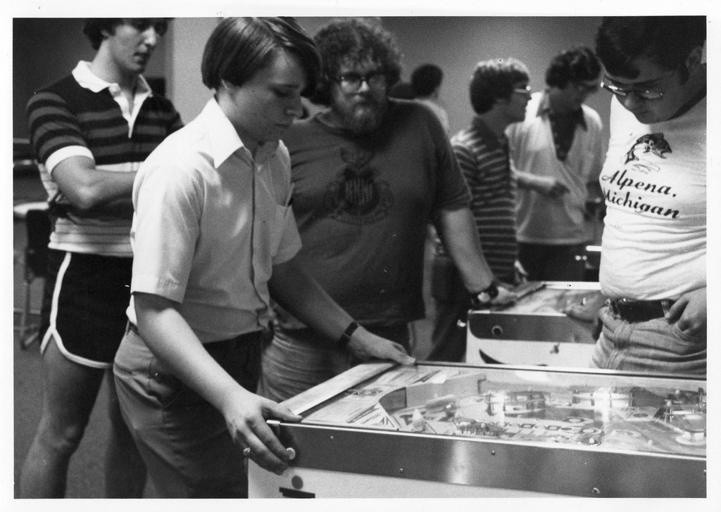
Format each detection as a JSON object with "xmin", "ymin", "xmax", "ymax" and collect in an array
[
  {"xmin": 330, "ymin": 71, "xmax": 388, "ymax": 94},
  {"xmin": 514, "ymin": 86, "xmax": 533, "ymax": 98},
  {"xmin": 601, "ymin": 73, "xmax": 678, "ymax": 100}
]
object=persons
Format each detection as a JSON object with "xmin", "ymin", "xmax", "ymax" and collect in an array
[
  {"xmin": 505, "ymin": 46, "xmax": 610, "ymax": 283},
  {"xmin": 260, "ymin": 17, "xmax": 521, "ymax": 399},
  {"xmin": 408, "ymin": 63, "xmax": 452, "ymax": 140},
  {"xmin": 18, "ymin": 19, "xmax": 187, "ymax": 498},
  {"xmin": 113, "ymin": 18, "xmax": 417, "ymax": 498},
  {"xmin": 560, "ymin": 17, "xmax": 708, "ymax": 379},
  {"xmin": 422, "ymin": 55, "xmax": 534, "ymax": 364}
]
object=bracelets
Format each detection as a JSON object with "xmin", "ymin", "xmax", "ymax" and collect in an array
[{"xmin": 335, "ymin": 318, "xmax": 361, "ymax": 348}]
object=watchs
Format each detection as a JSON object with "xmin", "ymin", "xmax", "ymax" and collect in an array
[{"xmin": 467, "ymin": 279, "xmax": 503, "ymax": 306}]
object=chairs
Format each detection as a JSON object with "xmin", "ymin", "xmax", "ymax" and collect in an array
[{"xmin": 18, "ymin": 209, "xmax": 51, "ymax": 350}]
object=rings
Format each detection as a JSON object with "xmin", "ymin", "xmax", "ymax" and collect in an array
[
  {"xmin": 580, "ymin": 296, "xmax": 590, "ymax": 304},
  {"xmin": 242, "ymin": 447, "xmax": 253, "ymax": 456}
]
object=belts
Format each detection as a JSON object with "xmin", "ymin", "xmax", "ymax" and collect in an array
[{"xmin": 608, "ymin": 297, "xmax": 677, "ymax": 324}]
